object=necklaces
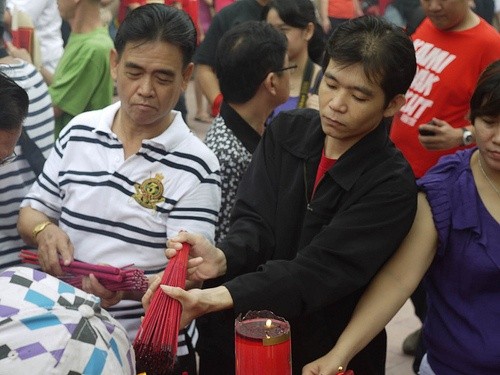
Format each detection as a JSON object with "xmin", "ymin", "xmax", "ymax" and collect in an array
[{"xmin": 477, "ymin": 154, "xmax": 500, "ymax": 195}]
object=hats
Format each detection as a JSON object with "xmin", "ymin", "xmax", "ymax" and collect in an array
[{"xmin": 0, "ymin": 266, "xmax": 136, "ymax": 375}]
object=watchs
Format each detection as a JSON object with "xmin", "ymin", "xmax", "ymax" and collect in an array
[{"xmin": 460, "ymin": 127, "xmax": 473, "ymax": 147}]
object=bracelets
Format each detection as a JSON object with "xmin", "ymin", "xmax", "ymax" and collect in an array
[{"xmin": 32, "ymin": 221, "xmax": 52, "ymax": 245}]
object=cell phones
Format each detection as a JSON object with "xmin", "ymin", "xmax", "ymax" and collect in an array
[{"xmin": 419, "ymin": 122, "xmax": 436, "ymax": 136}]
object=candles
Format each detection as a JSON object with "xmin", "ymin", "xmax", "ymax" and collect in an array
[{"xmin": 235, "ymin": 310, "xmax": 292, "ymax": 375}]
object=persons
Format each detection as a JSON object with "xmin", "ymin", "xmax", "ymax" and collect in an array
[
  {"xmin": 390, "ymin": 0, "xmax": 500, "ymax": 354},
  {"xmin": 141, "ymin": 15, "xmax": 419, "ymax": 375},
  {"xmin": 0, "ymin": 19, "xmax": 55, "ymax": 271},
  {"xmin": 0, "ymin": 0, "xmax": 427, "ymax": 141},
  {"xmin": 16, "ymin": 3, "xmax": 222, "ymax": 375},
  {"xmin": 303, "ymin": 58, "xmax": 500, "ymax": 375},
  {"xmin": 194, "ymin": 19, "xmax": 297, "ymax": 374}
]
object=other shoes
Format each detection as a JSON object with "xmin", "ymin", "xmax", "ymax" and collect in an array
[{"xmin": 403, "ymin": 328, "xmax": 422, "ymax": 356}]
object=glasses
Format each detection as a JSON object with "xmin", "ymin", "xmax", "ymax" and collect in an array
[
  {"xmin": 261, "ymin": 63, "xmax": 298, "ymax": 83},
  {"xmin": 0, "ymin": 151, "xmax": 17, "ymax": 167}
]
